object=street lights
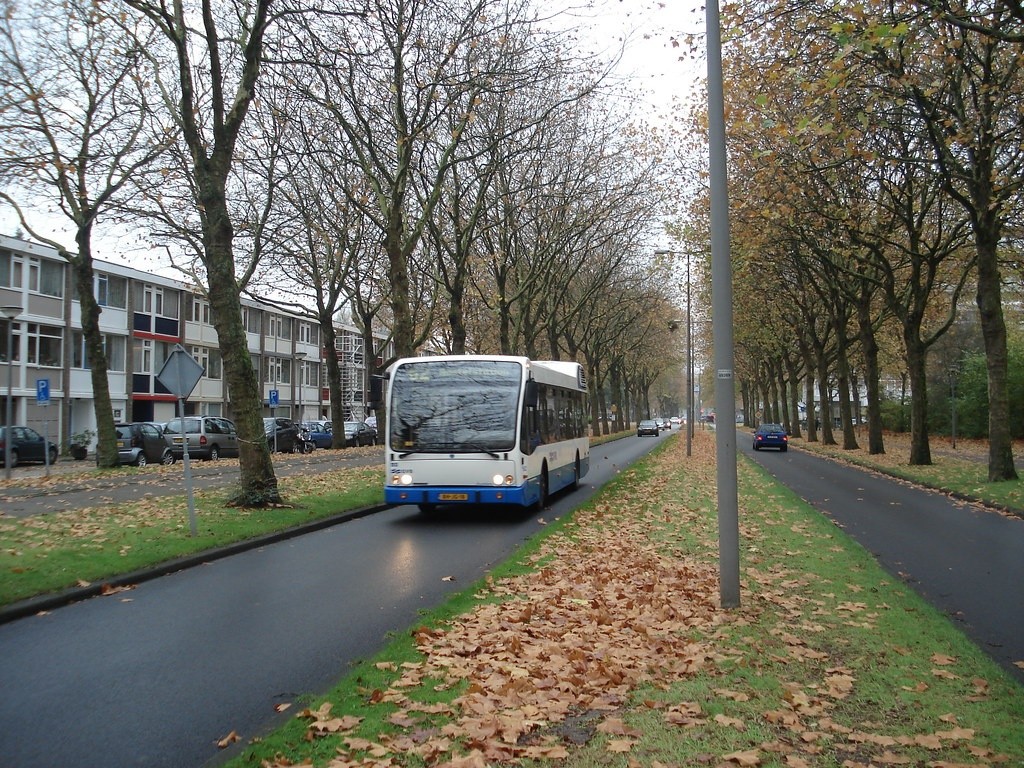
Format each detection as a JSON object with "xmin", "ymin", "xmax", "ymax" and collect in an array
[
  {"xmin": 654, "ymin": 246, "xmax": 711, "ymax": 456},
  {"xmin": 672, "ymin": 319, "xmax": 714, "ymax": 438}
]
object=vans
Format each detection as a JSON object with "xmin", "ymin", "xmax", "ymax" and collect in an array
[{"xmin": 162, "ymin": 415, "xmax": 241, "ymax": 460}]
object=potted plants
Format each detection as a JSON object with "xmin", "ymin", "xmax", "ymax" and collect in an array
[{"xmin": 64, "ymin": 429, "xmax": 96, "ymax": 460}]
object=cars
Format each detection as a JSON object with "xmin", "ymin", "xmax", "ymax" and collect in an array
[
  {"xmin": 751, "ymin": 423, "xmax": 789, "ymax": 452},
  {"xmin": 0, "ymin": 425, "xmax": 59, "ymax": 467},
  {"xmin": 262, "ymin": 416, "xmax": 378, "ymax": 454},
  {"xmin": 96, "ymin": 422, "xmax": 176, "ymax": 467},
  {"xmin": 636, "ymin": 416, "xmax": 687, "ymax": 437},
  {"xmin": 697, "ymin": 415, "xmax": 707, "ymax": 424}
]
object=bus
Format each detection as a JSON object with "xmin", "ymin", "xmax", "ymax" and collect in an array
[{"xmin": 371, "ymin": 354, "xmax": 590, "ymax": 514}]
